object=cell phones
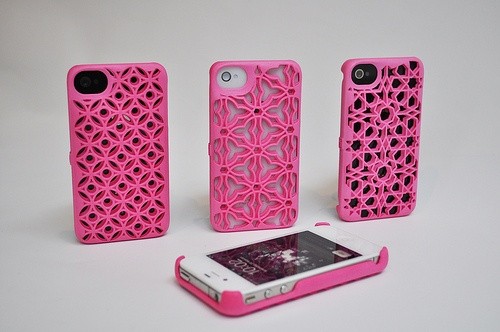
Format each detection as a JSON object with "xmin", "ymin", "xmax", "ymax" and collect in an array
[
  {"xmin": 175, "ymin": 222, "xmax": 388, "ymax": 316},
  {"xmin": 67, "ymin": 63, "xmax": 170, "ymax": 244},
  {"xmin": 336, "ymin": 57, "xmax": 424, "ymax": 222},
  {"xmin": 208, "ymin": 59, "xmax": 303, "ymax": 234}
]
